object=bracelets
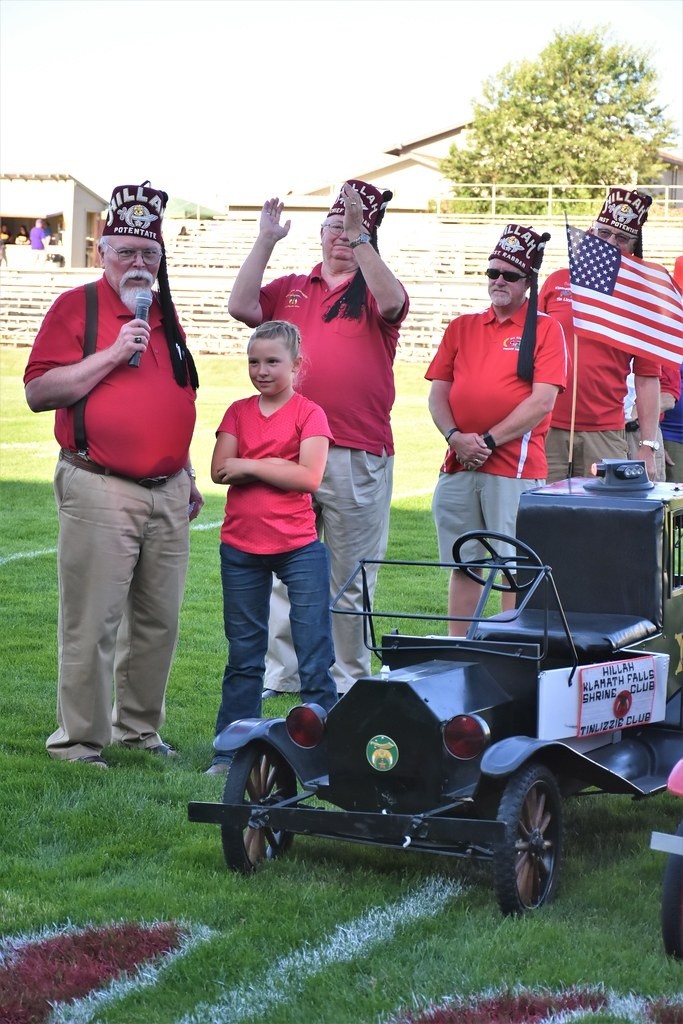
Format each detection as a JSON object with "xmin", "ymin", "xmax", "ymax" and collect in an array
[
  {"xmin": 187, "ymin": 469, "xmax": 196, "ymax": 480},
  {"xmin": 445, "ymin": 428, "xmax": 460, "ymax": 442}
]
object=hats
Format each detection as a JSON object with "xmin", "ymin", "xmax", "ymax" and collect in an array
[
  {"xmin": 102, "ymin": 185, "xmax": 164, "ymax": 244},
  {"xmin": 36, "ymin": 219, "xmax": 44, "ymax": 222},
  {"xmin": 327, "ymin": 180, "xmax": 393, "ymax": 233},
  {"xmin": 489, "ymin": 224, "xmax": 550, "ymax": 275},
  {"xmin": 596, "ymin": 189, "xmax": 652, "ymax": 237}
]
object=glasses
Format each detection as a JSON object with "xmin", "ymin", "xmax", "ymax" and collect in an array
[
  {"xmin": 321, "ymin": 223, "xmax": 370, "ymax": 239},
  {"xmin": 593, "ymin": 227, "xmax": 639, "ymax": 244},
  {"xmin": 486, "ymin": 269, "xmax": 528, "ymax": 282},
  {"xmin": 107, "ymin": 244, "xmax": 163, "ymax": 265}
]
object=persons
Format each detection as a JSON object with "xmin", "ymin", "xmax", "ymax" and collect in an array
[
  {"xmin": 423, "ymin": 225, "xmax": 568, "ymax": 638},
  {"xmin": 227, "ymin": 180, "xmax": 410, "ymax": 702},
  {"xmin": 23, "ymin": 186, "xmax": 205, "ymax": 773},
  {"xmin": 537, "ymin": 185, "xmax": 683, "ymax": 486},
  {"xmin": 204, "ymin": 319, "xmax": 340, "ymax": 779},
  {"xmin": 0, "ymin": 219, "xmax": 64, "ymax": 269}
]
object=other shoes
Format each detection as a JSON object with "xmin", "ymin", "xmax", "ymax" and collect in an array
[
  {"xmin": 78, "ymin": 756, "xmax": 109, "ymax": 767},
  {"xmin": 204, "ymin": 764, "xmax": 230, "ymax": 774},
  {"xmin": 145, "ymin": 742, "xmax": 176, "ymax": 757}
]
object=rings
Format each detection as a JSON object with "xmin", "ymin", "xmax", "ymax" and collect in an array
[
  {"xmin": 134, "ymin": 336, "xmax": 141, "ymax": 343},
  {"xmin": 267, "ymin": 212, "xmax": 271, "ymax": 214},
  {"xmin": 351, "ymin": 202, "xmax": 356, "ymax": 204},
  {"xmin": 474, "ymin": 459, "xmax": 478, "ymax": 463}
]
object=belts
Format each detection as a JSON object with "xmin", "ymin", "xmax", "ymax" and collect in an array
[{"xmin": 63, "ymin": 449, "xmax": 183, "ymax": 489}]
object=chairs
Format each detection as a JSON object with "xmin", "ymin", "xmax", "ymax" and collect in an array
[{"xmin": 466, "ymin": 495, "xmax": 666, "ymax": 659}]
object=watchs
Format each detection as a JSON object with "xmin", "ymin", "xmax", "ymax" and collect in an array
[
  {"xmin": 483, "ymin": 431, "xmax": 496, "ymax": 449},
  {"xmin": 639, "ymin": 441, "xmax": 660, "ymax": 451},
  {"xmin": 349, "ymin": 232, "xmax": 370, "ymax": 248}
]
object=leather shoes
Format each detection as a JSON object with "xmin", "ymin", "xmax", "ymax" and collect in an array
[{"xmin": 262, "ymin": 689, "xmax": 280, "ymax": 699}]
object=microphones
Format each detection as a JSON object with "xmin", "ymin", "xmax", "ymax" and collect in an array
[{"xmin": 128, "ymin": 290, "xmax": 153, "ymax": 368}]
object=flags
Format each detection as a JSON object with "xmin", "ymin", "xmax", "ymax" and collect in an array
[{"xmin": 566, "ymin": 222, "xmax": 683, "ymax": 371}]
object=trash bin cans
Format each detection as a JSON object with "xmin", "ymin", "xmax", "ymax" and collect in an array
[{"xmin": 46, "ymin": 254, "xmax": 63, "ymax": 269}]
